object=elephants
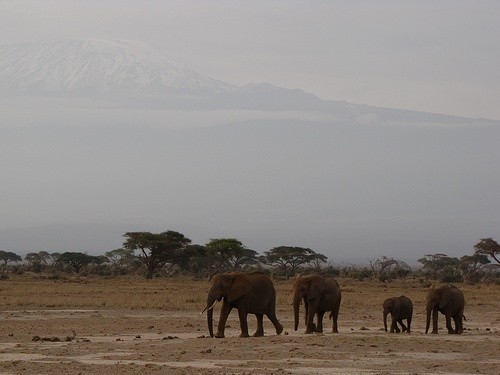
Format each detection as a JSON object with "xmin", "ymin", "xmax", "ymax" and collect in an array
[
  {"xmin": 425, "ymin": 284, "xmax": 466, "ymax": 334},
  {"xmin": 292, "ymin": 273, "xmax": 341, "ymax": 333},
  {"xmin": 199, "ymin": 269, "xmax": 284, "ymax": 338},
  {"xmin": 382, "ymin": 294, "xmax": 413, "ymax": 333}
]
